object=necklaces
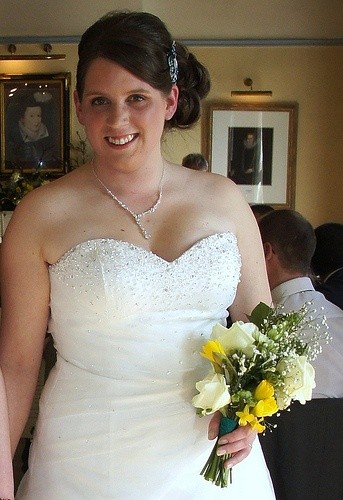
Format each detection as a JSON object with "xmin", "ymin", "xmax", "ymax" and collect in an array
[{"xmin": 91, "ymin": 158, "xmax": 165, "ymax": 240}]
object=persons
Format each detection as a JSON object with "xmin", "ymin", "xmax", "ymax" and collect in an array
[
  {"xmin": 226, "ymin": 204, "xmax": 343, "ymax": 401},
  {"xmin": 182, "ymin": 152, "xmax": 208, "ymax": 172},
  {"xmin": 1, "ymin": 12, "xmax": 277, "ymax": 500}
]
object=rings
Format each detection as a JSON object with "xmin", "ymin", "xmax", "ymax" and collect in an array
[{"xmin": 248, "ymin": 443, "xmax": 252, "ymax": 448}]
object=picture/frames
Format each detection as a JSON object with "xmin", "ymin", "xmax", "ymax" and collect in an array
[
  {"xmin": 208, "ymin": 101, "xmax": 299, "ymax": 211},
  {"xmin": 0, "ymin": 72, "xmax": 71, "ymax": 179}
]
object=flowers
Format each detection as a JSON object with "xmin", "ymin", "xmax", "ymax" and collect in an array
[
  {"xmin": 191, "ymin": 290, "xmax": 332, "ymax": 488},
  {"xmin": 0, "ymin": 170, "xmax": 52, "ymax": 206}
]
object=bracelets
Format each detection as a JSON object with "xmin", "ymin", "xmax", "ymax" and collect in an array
[{"xmin": 0, "ymin": 497, "xmax": 12, "ymax": 500}]
